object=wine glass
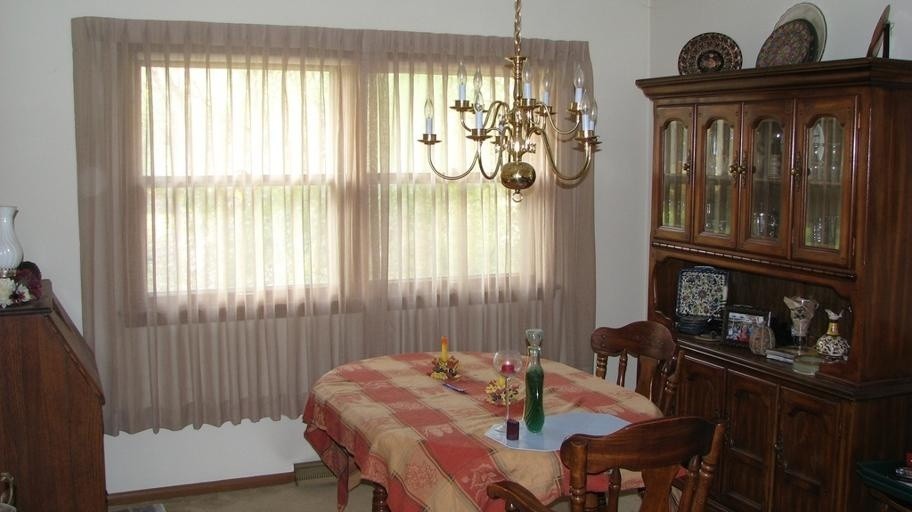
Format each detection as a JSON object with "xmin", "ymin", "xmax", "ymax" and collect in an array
[{"xmin": 492, "ymin": 350, "xmax": 523, "ymax": 433}]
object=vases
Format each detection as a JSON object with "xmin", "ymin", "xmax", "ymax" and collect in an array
[{"xmin": 1, "ymin": 204, "xmax": 24, "ymax": 270}]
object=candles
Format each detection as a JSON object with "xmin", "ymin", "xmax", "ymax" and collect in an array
[{"xmin": 501, "ymin": 360, "xmax": 515, "ymax": 373}]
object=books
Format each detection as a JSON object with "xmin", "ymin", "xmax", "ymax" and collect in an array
[{"xmin": 765, "ymin": 344, "xmax": 848, "ymax": 384}]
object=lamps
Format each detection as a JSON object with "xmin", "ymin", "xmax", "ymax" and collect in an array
[{"xmin": 417, "ymin": 0, "xmax": 603, "ymax": 201}]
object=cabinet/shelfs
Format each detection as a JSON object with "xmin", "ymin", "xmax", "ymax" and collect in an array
[{"xmin": 635, "ymin": 57, "xmax": 912, "ymax": 511}]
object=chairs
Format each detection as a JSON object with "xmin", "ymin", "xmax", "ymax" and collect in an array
[
  {"xmin": 591, "ymin": 321, "xmax": 686, "ymax": 413},
  {"xmin": 486, "ymin": 416, "xmax": 725, "ymax": 511}
]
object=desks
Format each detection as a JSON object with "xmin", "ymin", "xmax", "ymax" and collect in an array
[
  {"xmin": 303, "ymin": 348, "xmax": 663, "ymax": 512},
  {"xmin": 0, "ymin": 276, "xmax": 108, "ymax": 510}
]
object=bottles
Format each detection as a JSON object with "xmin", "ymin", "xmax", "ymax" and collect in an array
[{"xmin": 521, "ymin": 328, "xmax": 546, "ymax": 434}]
